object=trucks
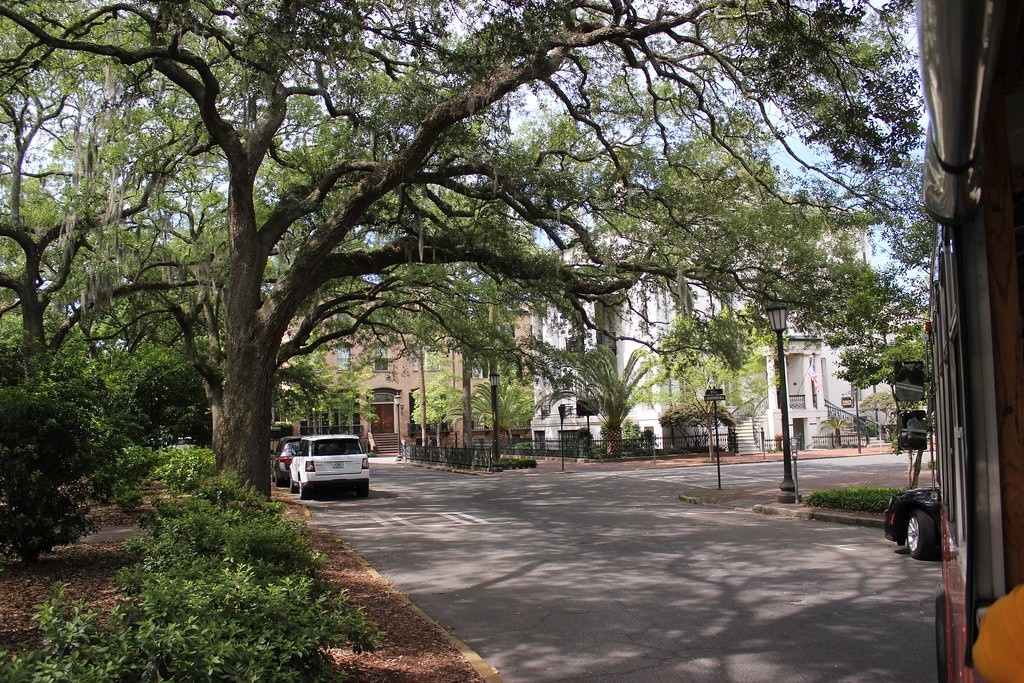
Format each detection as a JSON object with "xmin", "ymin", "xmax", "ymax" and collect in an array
[{"xmin": 892, "ymin": 0, "xmax": 1024, "ymax": 683}]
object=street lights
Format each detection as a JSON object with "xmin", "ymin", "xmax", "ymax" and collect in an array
[
  {"xmin": 489, "ymin": 372, "xmax": 503, "ymax": 472},
  {"xmin": 767, "ymin": 290, "xmax": 802, "ymax": 503},
  {"xmin": 394, "ymin": 392, "xmax": 403, "ymax": 461}
]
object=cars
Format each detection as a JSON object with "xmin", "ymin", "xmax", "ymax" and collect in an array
[
  {"xmin": 884, "ymin": 487, "xmax": 943, "ymax": 560},
  {"xmin": 272, "ymin": 436, "xmax": 303, "ymax": 486},
  {"xmin": 289, "ymin": 434, "xmax": 370, "ymax": 499}
]
